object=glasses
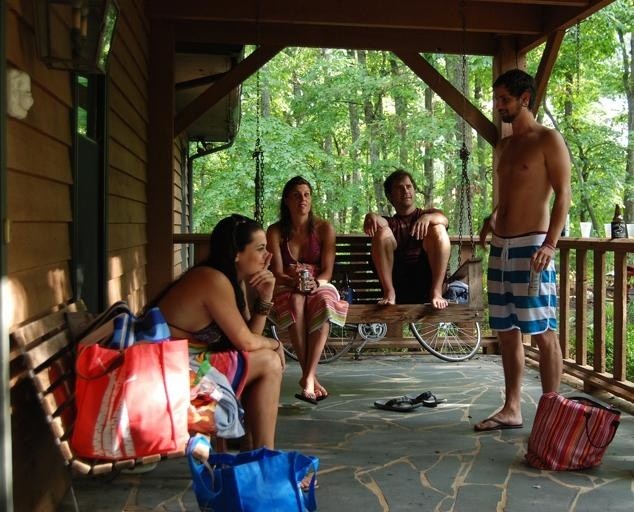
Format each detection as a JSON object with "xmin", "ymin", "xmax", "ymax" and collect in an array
[{"xmin": 230, "ymin": 213, "xmax": 250, "ymax": 248}]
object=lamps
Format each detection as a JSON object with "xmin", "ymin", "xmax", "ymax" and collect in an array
[{"xmin": 32, "ymin": 1, "xmax": 120, "ymax": 75}]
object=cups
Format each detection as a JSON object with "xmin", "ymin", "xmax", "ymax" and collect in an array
[
  {"xmin": 604, "ymin": 223, "xmax": 611, "ymax": 238},
  {"xmin": 580, "ymin": 221, "xmax": 592, "ymax": 239},
  {"xmin": 626, "ymin": 224, "xmax": 634, "ymax": 239}
]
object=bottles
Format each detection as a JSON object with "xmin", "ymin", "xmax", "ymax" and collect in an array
[
  {"xmin": 339, "ymin": 271, "xmax": 351, "ymax": 304},
  {"xmin": 529, "ymin": 255, "xmax": 543, "ymax": 298},
  {"xmin": 611, "ymin": 203, "xmax": 626, "ymax": 238}
]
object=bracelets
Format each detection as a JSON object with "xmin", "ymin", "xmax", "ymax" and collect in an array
[
  {"xmin": 314, "ymin": 276, "xmax": 319, "ymax": 290},
  {"xmin": 255, "ymin": 298, "xmax": 274, "ymax": 316},
  {"xmin": 541, "ymin": 241, "xmax": 555, "ymax": 252}
]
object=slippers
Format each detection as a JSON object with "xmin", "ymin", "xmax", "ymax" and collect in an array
[
  {"xmin": 294, "ymin": 390, "xmax": 317, "ymax": 405},
  {"xmin": 372, "ymin": 395, "xmax": 423, "ymax": 412},
  {"xmin": 406, "ymin": 390, "xmax": 448, "ymax": 408},
  {"xmin": 315, "ymin": 386, "xmax": 327, "ymax": 401},
  {"xmin": 472, "ymin": 412, "xmax": 522, "ymax": 433}
]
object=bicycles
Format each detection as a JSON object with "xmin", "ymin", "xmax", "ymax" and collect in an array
[{"xmin": 270, "ymin": 284, "xmax": 482, "ymax": 364}]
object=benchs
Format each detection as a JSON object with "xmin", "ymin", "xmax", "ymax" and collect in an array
[
  {"xmin": 264, "ymin": 236, "xmax": 486, "ymax": 326},
  {"xmin": 13, "ymin": 298, "xmax": 212, "ymax": 511}
]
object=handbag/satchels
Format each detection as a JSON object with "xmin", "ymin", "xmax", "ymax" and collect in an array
[
  {"xmin": 65, "ymin": 330, "xmax": 191, "ymax": 462},
  {"xmin": 524, "ymin": 387, "xmax": 621, "ymax": 474},
  {"xmin": 183, "ymin": 431, "xmax": 320, "ymax": 512}
]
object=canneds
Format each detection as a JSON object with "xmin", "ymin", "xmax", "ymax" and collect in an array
[
  {"xmin": 526, "ymin": 262, "xmax": 542, "ymax": 297},
  {"xmin": 297, "ymin": 269, "xmax": 311, "ymax": 293}
]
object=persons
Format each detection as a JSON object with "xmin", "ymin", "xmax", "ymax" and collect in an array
[
  {"xmin": 362, "ymin": 170, "xmax": 453, "ymax": 309},
  {"xmin": 144, "ymin": 213, "xmax": 287, "ymax": 454},
  {"xmin": 262, "ymin": 175, "xmax": 337, "ymax": 404},
  {"xmin": 474, "ymin": 69, "xmax": 571, "ymax": 431}
]
